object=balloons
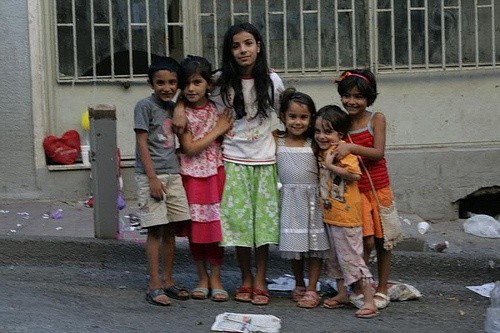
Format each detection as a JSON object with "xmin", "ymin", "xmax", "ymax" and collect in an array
[{"xmin": 81, "ymin": 111, "xmax": 90, "ymax": 132}]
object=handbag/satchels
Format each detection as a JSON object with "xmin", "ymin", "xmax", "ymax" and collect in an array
[
  {"xmin": 378, "ymin": 199, "xmax": 404, "ymax": 249},
  {"xmin": 42, "ymin": 130, "xmax": 81, "ymax": 165}
]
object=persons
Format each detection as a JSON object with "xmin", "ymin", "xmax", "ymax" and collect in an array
[
  {"xmin": 311, "ymin": 69, "xmax": 404, "ymax": 308},
  {"xmin": 177, "ymin": 55, "xmax": 233, "ymax": 301},
  {"xmin": 171, "ymin": 22, "xmax": 285, "ymax": 305},
  {"xmin": 314, "ymin": 105, "xmax": 380, "ymax": 317},
  {"xmin": 272, "ymin": 87, "xmax": 330, "ymax": 309},
  {"xmin": 133, "ymin": 57, "xmax": 190, "ymax": 307}
]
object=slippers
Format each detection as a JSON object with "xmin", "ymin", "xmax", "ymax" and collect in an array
[
  {"xmin": 211, "ymin": 289, "xmax": 229, "ymax": 301},
  {"xmin": 164, "ymin": 286, "xmax": 189, "ymax": 301},
  {"xmin": 144, "ymin": 288, "xmax": 172, "ymax": 306},
  {"xmin": 356, "ymin": 303, "xmax": 381, "ymax": 318},
  {"xmin": 233, "ymin": 284, "xmax": 253, "ymax": 301},
  {"xmin": 251, "ymin": 288, "xmax": 270, "ymax": 304},
  {"xmin": 298, "ymin": 289, "xmax": 321, "ymax": 308},
  {"xmin": 292, "ymin": 285, "xmax": 306, "ymax": 301},
  {"xmin": 323, "ymin": 297, "xmax": 349, "ymax": 308},
  {"xmin": 191, "ymin": 287, "xmax": 209, "ymax": 298}
]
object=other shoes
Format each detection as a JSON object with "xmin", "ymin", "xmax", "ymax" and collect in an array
[
  {"xmin": 374, "ymin": 292, "xmax": 391, "ymax": 308},
  {"xmin": 348, "ymin": 294, "xmax": 366, "ymax": 308}
]
